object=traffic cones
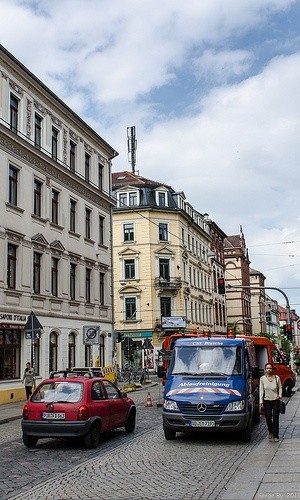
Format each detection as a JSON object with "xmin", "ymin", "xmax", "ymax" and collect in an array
[{"xmin": 144, "ymin": 392, "xmax": 153, "ymax": 407}]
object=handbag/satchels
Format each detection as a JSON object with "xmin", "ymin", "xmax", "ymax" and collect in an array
[{"xmin": 280, "ymin": 401, "xmax": 286, "ymax": 414}]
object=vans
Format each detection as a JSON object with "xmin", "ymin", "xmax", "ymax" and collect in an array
[
  {"xmin": 156, "ymin": 328, "xmax": 296, "ymax": 397},
  {"xmin": 157, "ymin": 338, "xmax": 259, "ymax": 443}
]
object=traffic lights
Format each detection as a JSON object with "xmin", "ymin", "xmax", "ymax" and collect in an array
[
  {"xmin": 115, "ymin": 332, "xmax": 124, "ymax": 343},
  {"xmin": 279, "ymin": 325, "xmax": 287, "ymax": 336},
  {"xmin": 217, "ymin": 277, "xmax": 226, "ymax": 295},
  {"xmin": 287, "ymin": 325, "xmax": 292, "ymax": 340}
]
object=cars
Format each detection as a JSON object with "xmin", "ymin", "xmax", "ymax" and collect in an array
[{"xmin": 21, "ymin": 371, "xmax": 136, "ymax": 449}]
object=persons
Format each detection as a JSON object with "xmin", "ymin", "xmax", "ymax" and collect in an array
[
  {"xmin": 279, "ymin": 348, "xmax": 284, "ymax": 359},
  {"xmin": 259, "ymin": 363, "xmax": 282, "ymax": 442},
  {"xmin": 22, "ymin": 362, "xmax": 36, "ymax": 400},
  {"xmin": 294, "ymin": 351, "xmax": 300, "ymax": 376}
]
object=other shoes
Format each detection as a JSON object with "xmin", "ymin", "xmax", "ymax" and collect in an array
[
  {"xmin": 274, "ymin": 438, "xmax": 278, "ymax": 442},
  {"xmin": 269, "ymin": 432, "xmax": 272, "ymax": 439}
]
